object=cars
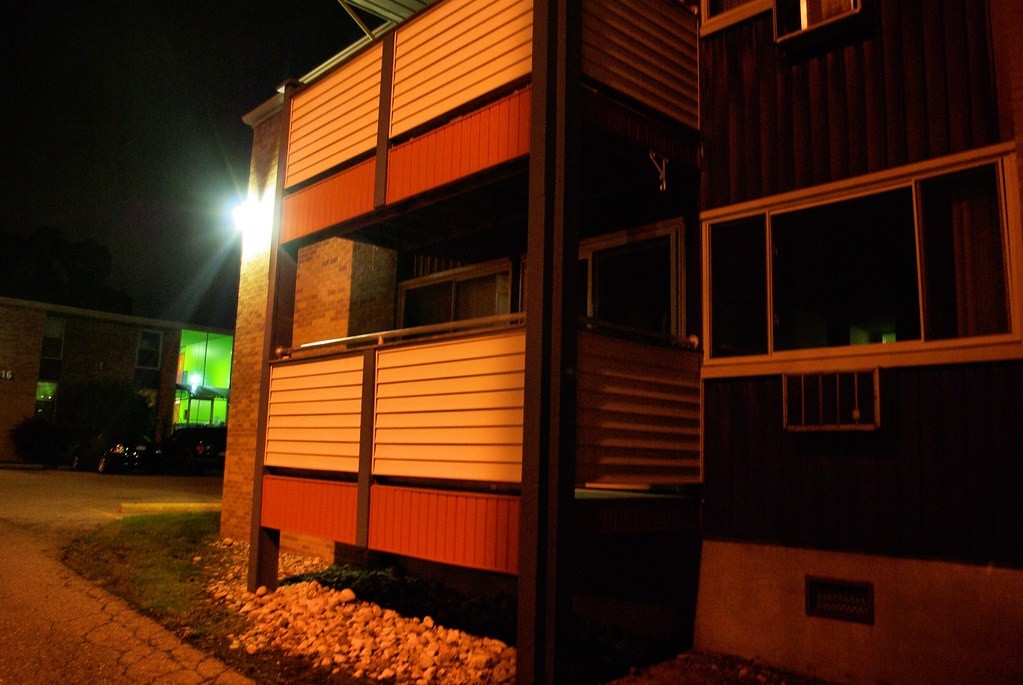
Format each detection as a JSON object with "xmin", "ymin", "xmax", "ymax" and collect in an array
[
  {"xmin": 162, "ymin": 427, "xmax": 227, "ymax": 474},
  {"xmin": 72, "ymin": 430, "xmax": 163, "ymax": 475}
]
